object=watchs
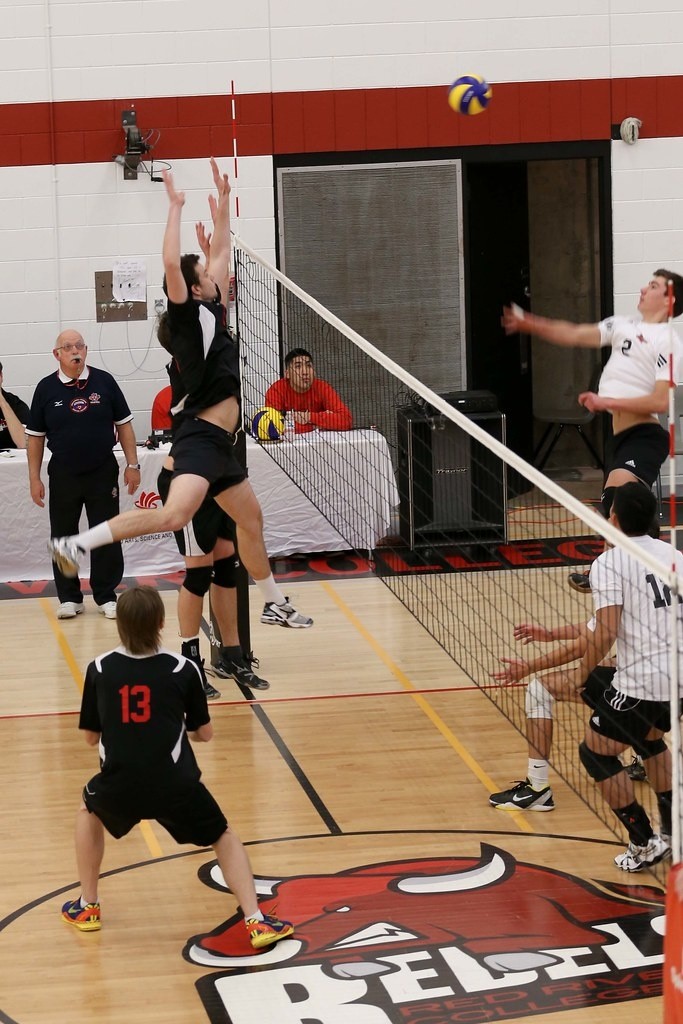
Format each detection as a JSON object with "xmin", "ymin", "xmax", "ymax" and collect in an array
[{"xmin": 127, "ymin": 464, "xmax": 140, "ymax": 470}]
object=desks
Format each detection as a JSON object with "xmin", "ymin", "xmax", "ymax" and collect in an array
[{"xmin": 0, "ymin": 431, "xmax": 402, "ymax": 580}]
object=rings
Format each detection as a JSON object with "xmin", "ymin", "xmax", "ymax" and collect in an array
[{"xmin": 136, "ymin": 485, "xmax": 139, "ymax": 486}]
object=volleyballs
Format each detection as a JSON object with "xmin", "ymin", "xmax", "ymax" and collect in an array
[
  {"xmin": 249, "ymin": 406, "xmax": 286, "ymax": 441},
  {"xmin": 448, "ymin": 74, "xmax": 493, "ymax": 115}
]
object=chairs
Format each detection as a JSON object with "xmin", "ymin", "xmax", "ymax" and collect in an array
[{"xmin": 522, "ymin": 362, "xmax": 604, "ymax": 492}]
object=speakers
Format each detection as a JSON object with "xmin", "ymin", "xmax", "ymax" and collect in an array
[{"xmin": 397, "ymin": 407, "xmax": 508, "ymax": 550}]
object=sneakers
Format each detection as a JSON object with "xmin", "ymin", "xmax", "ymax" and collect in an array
[
  {"xmin": 193, "ymin": 658, "xmax": 222, "ymax": 700},
  {"xmin": 260, "ymin": 596, "xmax": 314, "ymax": 628},
  {"xmin": 622, "ymin": 755, "xmax": 647, "ymax": 782},
  {"xmin": 61, "ymin": 894, "xmax": 102, "ymax": 929},
  {"xmin": 211, "ymin": 650, "xmax": 270, "ymax": 691},
  {"xmin": 614, "ymin": 833, "xmax": 672, "ymax": 873},
  {"xmin": 659, "ymin": 832, "xmax": 672, "ymax": 849},
  {"xmin": 46, "ymin": 536, "xmax": 86, "ymax": 578},
  {"xmin": 488, "ymin": 777, "xmax": 556, "ymax": 812},
  {"xmin": 245, "ymin": 903, "xmax": 295, "ymax": 949},
  {"xmin": 568, "ymin": 572, "xmax": 593, "ymax": 593},
  {"xmin": 57, "ymin": 602, "xmax": 85, "ymax": 619},
  {"xmin": 97, "ymin": 601, "xmax": 117, "ymax": 619}
]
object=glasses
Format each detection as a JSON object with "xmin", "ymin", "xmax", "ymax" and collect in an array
[{"xmin": 55, "ymin": 342, "xmax": 85, "ymax": 352}]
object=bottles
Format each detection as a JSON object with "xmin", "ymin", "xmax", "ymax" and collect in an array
[{"xmin": 284, "ymin": 411, "xmax": 295, "ymax": 444}]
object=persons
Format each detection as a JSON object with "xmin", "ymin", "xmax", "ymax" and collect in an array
[
  {"xmin": 47, "ymin": 154, "xmax": 314, "ymax": 629},
  {"xmin": 0, "ymin": 362, "xmax": 30, "ymax": 449},
  {"xmin": 157, "ymin": 223, "xmax": 269, "ymax": 698},
  {"xmin": 489, "ymin": 481, "xmax": 683, "ymax": 870},
  {"xmin": 501, "ymin": 270, "xmax": 683, "ymax": 591},
  {"xmin": 151, "ymin": 385, "xmax": 173, "ymax": 430},
  {"xmin": 24, "ymin": 328, "xmax": 141, "ymax": 619},
  {"xmin": 264, "ymin": 348, "xmax": 353, "ymax": 559},
  {"xmin": 59, "ymin": 586, "xmax": 294, "ymax": 949}
]
